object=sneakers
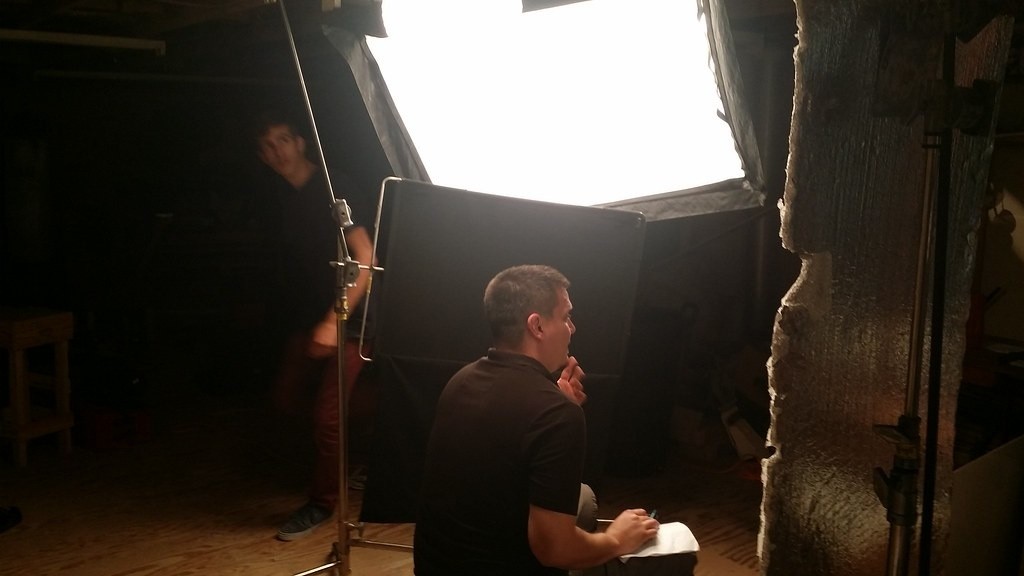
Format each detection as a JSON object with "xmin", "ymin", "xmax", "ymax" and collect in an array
[{"xmin": 277, "ymin": 502, "xmax": 334, "ymax": 541}]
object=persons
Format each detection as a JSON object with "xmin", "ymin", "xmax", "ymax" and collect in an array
[
  {"xmin": 255, "ymin": 112, "xmax": 379, "ymax": 541},
  {"xmin": 413, "ymin": 264, "xmax": 660, "ymax": 576}
]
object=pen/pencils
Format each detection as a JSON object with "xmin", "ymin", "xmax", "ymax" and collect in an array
[{"xmin": 595, "ymin": 517, "xmax": 614, "ymax": 523}]
